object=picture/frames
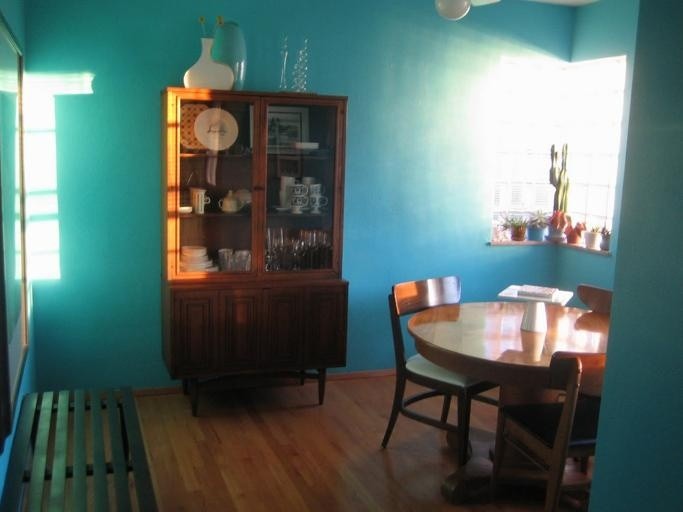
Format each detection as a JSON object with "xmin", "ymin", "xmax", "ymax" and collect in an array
[{"xmin": 247, "ymin": 101, "xmax": 311, "ymax": 156}]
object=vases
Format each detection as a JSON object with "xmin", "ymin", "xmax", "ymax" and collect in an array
[{"xmin": 184, "ymin": 38, "xmax": 235, "ymax": 91}]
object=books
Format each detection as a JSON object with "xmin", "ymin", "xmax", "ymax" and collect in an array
[{"xmin": 517, "ymin": 285, "xmax": 559, "ymax": 300}]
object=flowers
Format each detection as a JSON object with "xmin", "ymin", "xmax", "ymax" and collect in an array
[{"xmin": 199, "ymin": 15, "xmax": 223, "ymax": 39}]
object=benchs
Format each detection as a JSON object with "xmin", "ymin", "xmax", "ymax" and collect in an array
[{"xmin": 3, "ymin": 384, "xmax": 156, "ymax": 512}]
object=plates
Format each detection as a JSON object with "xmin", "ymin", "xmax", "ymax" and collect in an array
[
  {"xmin": 496, "ymin": 283, "xmax": 575, "ymax": 307},
  {"xmin": 275, "ymin": 207, "xmax": 290, "ymax": 212},
  {"xmin": 180, "ymin": 104, "xmax": 240, "ymax": 151}
]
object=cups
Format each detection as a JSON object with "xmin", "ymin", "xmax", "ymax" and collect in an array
[
  {"xmin": 519, "ymin": 301, "xmax": 548, "ymax": 334},
  {"xmin": 187, "ymin": 187, "xmax": 211, "ymax": 215},
  {"xmin": 217, "ymin": 247, "xmax": 252, "ymax": 273},
  {"xmin": 278, "ymin": 176, "xmax": 329, "ymax": 215}
]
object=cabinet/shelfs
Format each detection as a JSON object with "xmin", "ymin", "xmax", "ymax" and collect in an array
[{"xmin": 162, "ymin": 88, "xmax": 351, "ymax": 418}]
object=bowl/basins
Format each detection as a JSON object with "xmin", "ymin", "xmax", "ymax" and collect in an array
[
  {"xmin": 180, "ymin": 205, "xmax": 194, "ymax": 215},
  {"xmin": 180, "ymin": 245, "xmax": 220, "ymax": 273}
]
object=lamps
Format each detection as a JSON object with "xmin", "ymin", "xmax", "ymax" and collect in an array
[{"xmin": 435, "ymin": 0, "xmax": 471, "ymax": 21}]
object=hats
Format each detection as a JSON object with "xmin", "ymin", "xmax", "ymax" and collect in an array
[{"xmin": 194, "ymin": 108, "xmax": 239, "ymax": 150}]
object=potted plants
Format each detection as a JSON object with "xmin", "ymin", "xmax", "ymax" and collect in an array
[{"xmin": 497, "ymin": 141, "xmax": 611, "ymax": 251}]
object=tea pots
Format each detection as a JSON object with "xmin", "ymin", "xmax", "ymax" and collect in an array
[{"xmin": 218, "ymin": 190, "xmax": 245, "ymax": 214}]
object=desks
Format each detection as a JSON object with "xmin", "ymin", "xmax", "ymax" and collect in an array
[{"xmin": 407, "ymin": 295, "xmax": 613, "ymax": 473}]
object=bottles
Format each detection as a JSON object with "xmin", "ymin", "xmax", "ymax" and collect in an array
[
  {"xmin": 264, "ymin": 226, "xmax": 332, "ymax": 273},
  {"xmin": 279, "ymin": 26, "xmax": 315, "ymax": 94},
  {"xmin": 212, "ymin": 19, "xmax": 247, "ymax": 91}
]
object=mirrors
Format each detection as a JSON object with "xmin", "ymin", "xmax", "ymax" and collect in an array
[{"xmin": 0, "ymin": 14, "xmax": 32, "ymax": 440}]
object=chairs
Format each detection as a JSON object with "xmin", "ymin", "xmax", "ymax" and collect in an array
[
  {"xmin": 574, "ymin": 279, "xmax": 613, "ymax": 315},
  {"xmin": 383, "ymin": 276, "xmax": 499, "ymax": 478},
  {"xmin": 493, "ymin": 350, "xmax": 608, "ymax": 512}
]
